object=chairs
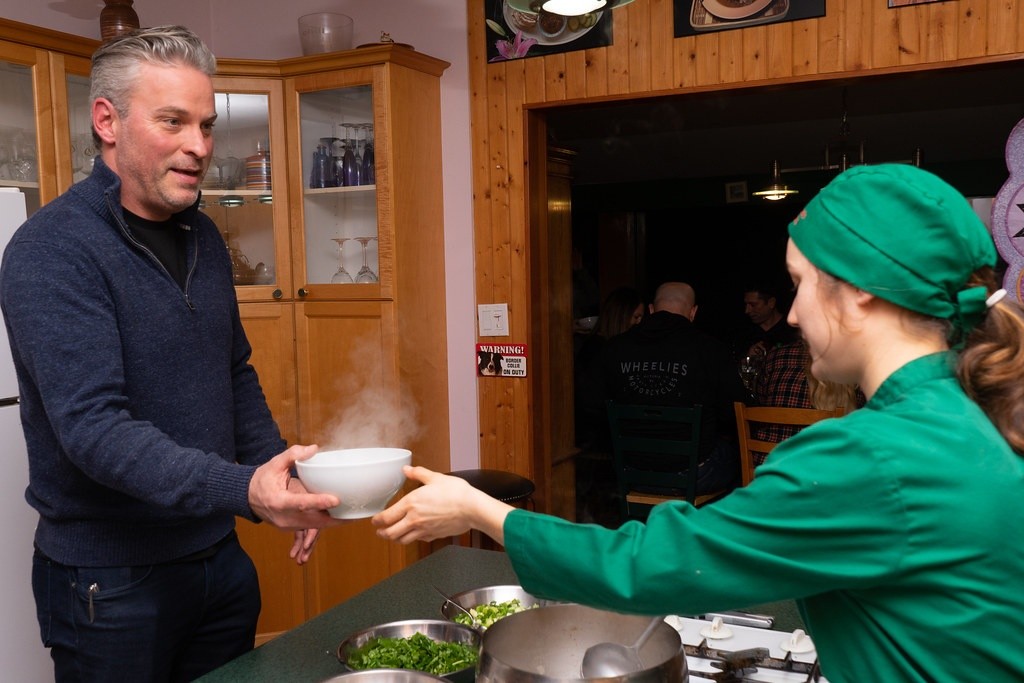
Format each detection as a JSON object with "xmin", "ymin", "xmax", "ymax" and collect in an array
[
  {"xmin": 604, "ymin": 396, "xmax": 728, "ymax": 525},
  {"xmin": 735, "ymin": 400, "xmax": 845, "ymax": 491}
]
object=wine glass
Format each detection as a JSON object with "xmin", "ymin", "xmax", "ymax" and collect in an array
[
  {"xmin": 737, "ymin": 356, "xmax": 758, "ymax": 389},
  {"xmin": 331, "ymin": 238, "xmax": 378, "ymax": 283}
]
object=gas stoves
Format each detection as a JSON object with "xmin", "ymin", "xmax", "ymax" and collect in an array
[{"xmin": 663, "ymin": 614, "xmax": 828, "ymax": 683}]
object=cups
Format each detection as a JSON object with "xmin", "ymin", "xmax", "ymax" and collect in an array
[
  {"xmin": 0, "ymin": 125, "xmax": 95, "ymax": 182},
  {"xmin": 537, "ymin": 11, "xmax": 567, "ymax": 37}
]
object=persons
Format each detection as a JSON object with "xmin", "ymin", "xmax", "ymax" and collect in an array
[
  {"xmin": 744, "ymin": 274, "xmax": 866, "ymax": 466},
  {"xmin": 574, "ymin": 282, "xmax": 736, "ymax": 494},
  {"xmin": 369, "ymin": 165, "xmax": 1023, "ymax": 681},
  {"xmin": 0, "ymin": 23, "xmax": 337, "ymax": 683}
]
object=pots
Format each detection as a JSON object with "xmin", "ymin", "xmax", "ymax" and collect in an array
[{"xmin": 477, "ymin": 601, "xmax": 690, "ymax": 683}]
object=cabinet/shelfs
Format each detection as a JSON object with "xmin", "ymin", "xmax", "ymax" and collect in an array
[{"xmin": 0, "ymin": 18, "xmax": 452, "ymax": 683}]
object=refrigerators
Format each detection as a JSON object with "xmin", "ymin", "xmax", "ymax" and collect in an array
[{"xmin": 0, "ymin": 187, "xmax": 55, "ymax": 683}]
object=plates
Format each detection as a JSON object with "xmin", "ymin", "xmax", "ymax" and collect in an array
[
  {"xmin": 700, "ymin": 0, "xmax": 773, "ymax": 19},
  {"xmin": 503, "ymin": 0, "xmax": 603, "ymax": 46}
]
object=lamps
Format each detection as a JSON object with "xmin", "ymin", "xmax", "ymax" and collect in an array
[{"xmin": 751, "ymin": 158, "xmax": 799, "ymax": 200}]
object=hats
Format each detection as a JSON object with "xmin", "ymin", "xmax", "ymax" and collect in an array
[{"xmin": 788, "ymin": 162, "xmax": 996, "ymax": 350}]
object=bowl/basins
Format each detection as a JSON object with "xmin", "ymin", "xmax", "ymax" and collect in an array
[
  {"xmin": 578, "ymin": 317, "xmax": 599, "ymax": 330},
  {"xmin": 297, "ymin": 12, "xmax": 354, "ymax": 56},
  {"xmin": 294, "ymin": 447, "xmax": 415, "ymax": 519},
  {"xmin": 320, "ymin": 584, "xmax": 569, "ymax": 683}
]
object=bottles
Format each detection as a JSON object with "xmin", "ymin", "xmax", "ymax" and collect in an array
[
  {"xmin": 100, "ymin": 0, "xmax": 139, "ymax": 43},
  {"xmin": 320, "ymin": 123, "xmax": 375, "ymax": 186},
  {"xmin": 246, "ymin": 142, "xmax": 272, "ymax": 191},
  {"xmin": 315, "ymin": 145, "xmax": 330, "ymax": 189}
]
